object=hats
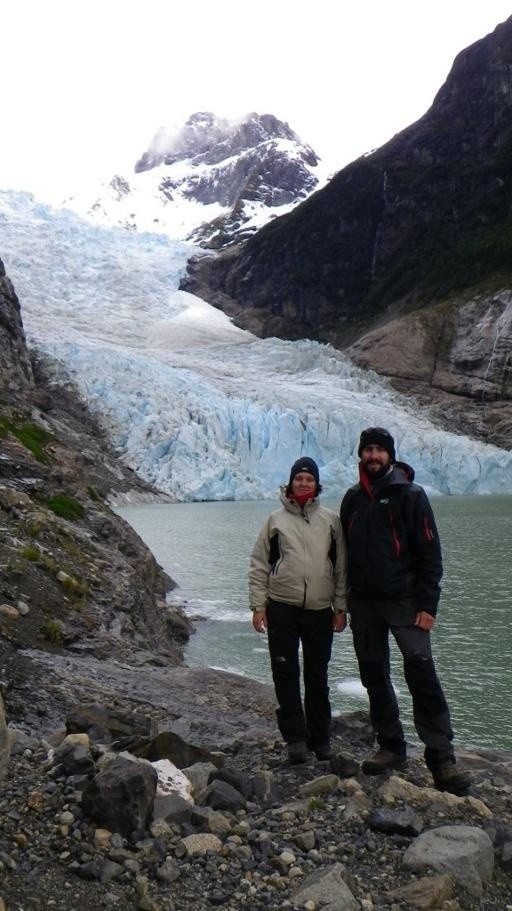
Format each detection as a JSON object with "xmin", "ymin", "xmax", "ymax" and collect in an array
[
  {"xmin": 290, "ymin": 456, "xmax": 319, "ymax": 486},
  {"xmin": 358, "ymin": 427, "xmax": 396, "ymax": 465}
]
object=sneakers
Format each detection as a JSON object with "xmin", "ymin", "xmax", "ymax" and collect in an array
[
  {"xmin": 287, "ymin": 740, "xmax": 307, "ymax": 765},
  {"xmin": 362, "ymin": 746, "xmax": 408, "ymax": 775},
  {"xmin": 430, "ymin": 760, "xmax": 472, "ymax": 793},
  {"xmin": 311, "ymin": 737, "xmax": 333, "ymax": 760}
]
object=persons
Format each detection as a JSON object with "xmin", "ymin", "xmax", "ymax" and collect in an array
[
  {"xmin": 246, "ymin": 457, "xmax": 349, "ymax": 766},
  {"xmin": 338, "ymin": 427, "xmax": 473, "ymax": 796}
]
